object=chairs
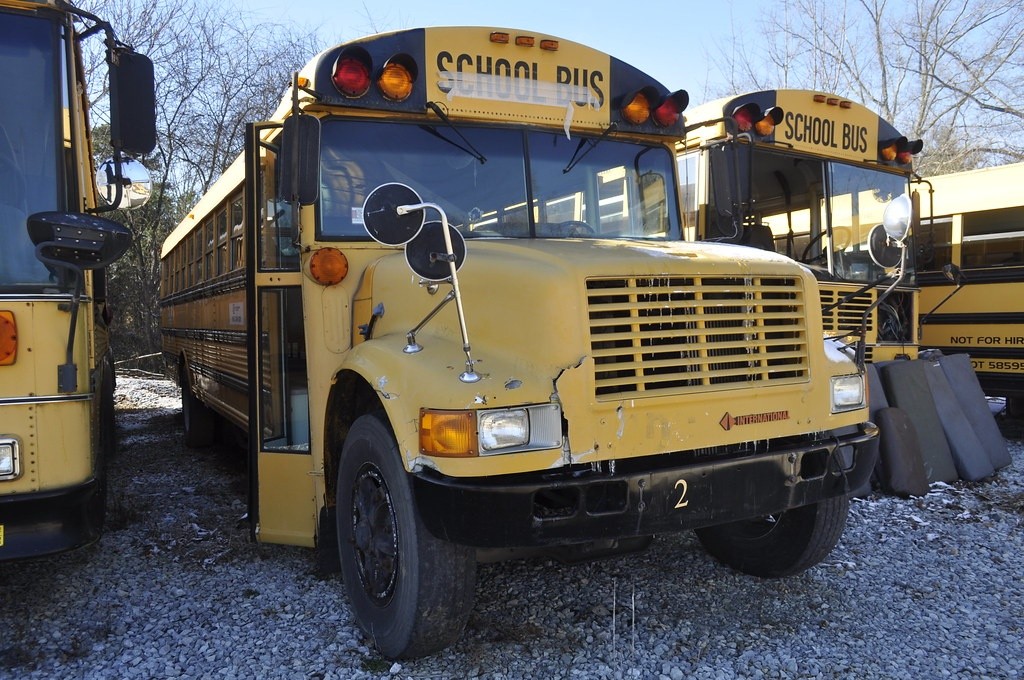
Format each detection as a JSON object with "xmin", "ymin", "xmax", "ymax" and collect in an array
[
  {"xmin": 1, "ymin": 205, "xmax": 25, "ymax": 257},
  {"xmin": 744, "ymin": 225, "xmax": 776, "ymax": 252},
  {"xmin": 963, "ymin": 261, "xmax": 984, "ymax": 279}
]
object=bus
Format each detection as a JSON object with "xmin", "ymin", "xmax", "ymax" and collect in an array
[
  {"xmin": 761, "ymin": 161, "xmax": 1024, "ymax": 419},
  {"xmin": 471, "ymin": 88, "xmax": 967, "ymax": 370},
  {"xmin": 0, "ymin": 0, "xmax": 161, "ymax": 568},
  {"xmin": 161, "ymin": 24, "xmax": 913, "ymax": 664}
]
